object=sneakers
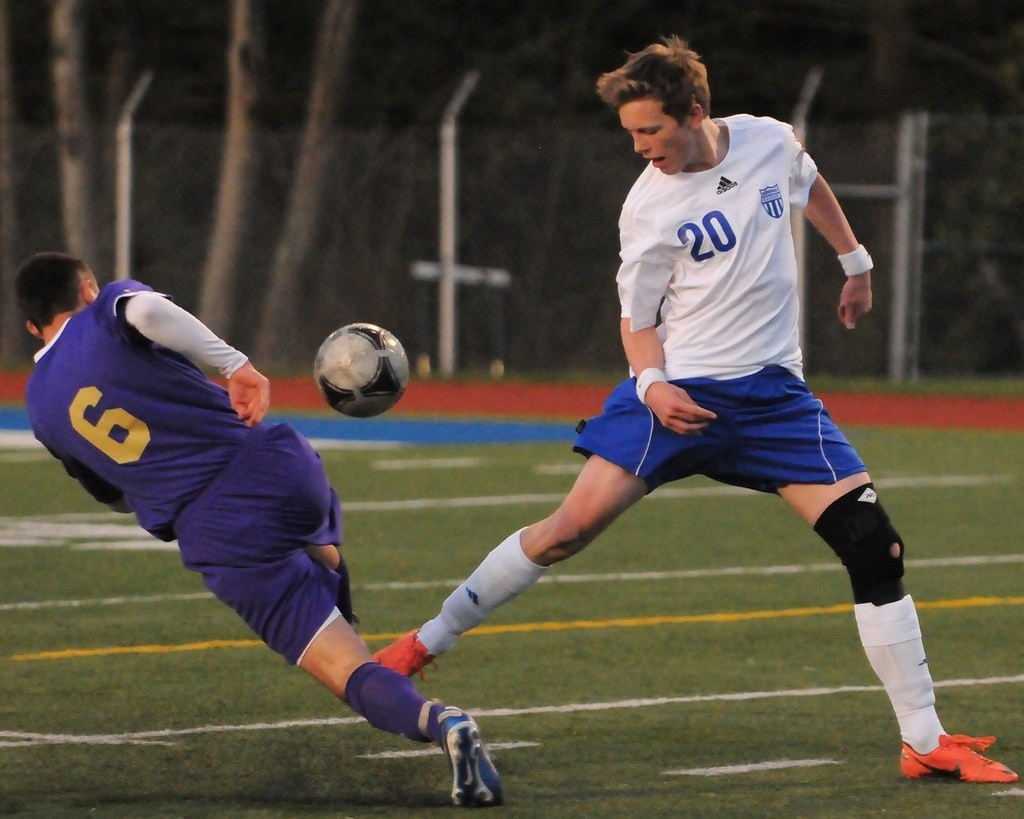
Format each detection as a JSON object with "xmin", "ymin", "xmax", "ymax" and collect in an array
[
  {"xmin": 344, "ymin": 612, "xmax": 360, "ymax": 633},
  {"xmin": 434, "ymin": 706, "xmax": 504, "ymax": 808},
  {"xmin": 372, "ymin": 629, "xmax": 439, "ymax": 682},
  {"xmin": 900, "ymin": 733, "xmax": 1019, "ymax": 783}
]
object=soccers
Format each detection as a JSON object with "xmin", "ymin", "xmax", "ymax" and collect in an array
[{"xmin": 312, "ymin": 322, "xmax": 409, "ymax": 419}]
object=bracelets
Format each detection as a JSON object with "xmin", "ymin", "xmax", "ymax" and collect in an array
[
  {"xmin": 837, "ymin": 243, "xmax": 874, "ymax": 277},
  {"xmin": 636, "ymin": 368, "xmax": 667, "ymax": 404}
]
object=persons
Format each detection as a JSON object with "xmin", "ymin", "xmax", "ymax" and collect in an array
[
  {"xmin": 12, "ymin": 254, "xmax": 504, "ymax": 808},
  {"xmin": 373, "ymin": 34, "xmax": 1019, "ymax": 783}
]
